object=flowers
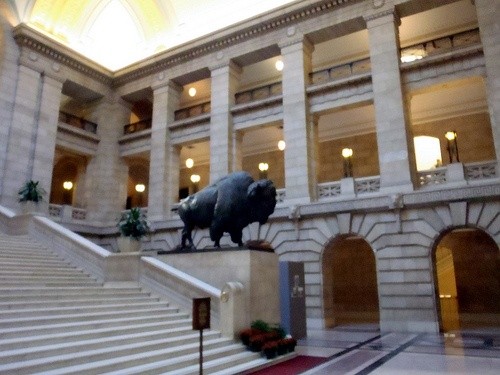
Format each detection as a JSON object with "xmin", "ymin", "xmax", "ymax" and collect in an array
[{"xmin": 239, "ymin": 320, "xmax": 296, "ymax": 349}]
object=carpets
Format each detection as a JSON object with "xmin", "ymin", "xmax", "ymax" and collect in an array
[{"xmin": 241, "ymin": 355, "xmax": 325, "ymax": 375}]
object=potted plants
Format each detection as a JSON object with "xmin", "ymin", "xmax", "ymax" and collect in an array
[
  {"xmin": 115, "ymin": 206, "xmax": 150, "ymax": 254},
  {"xmin": 18, "ymin": 180, "xmax": 44, "ymax": 212}
]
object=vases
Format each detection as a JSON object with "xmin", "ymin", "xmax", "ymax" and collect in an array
[{"xmin": 241, "ymin": 337, "xmax": 295, "ymax": 359}]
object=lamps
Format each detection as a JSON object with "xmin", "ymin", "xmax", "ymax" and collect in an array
[{"xmin": 186, "ymin": 146, "xmax": 194, "ymax": 168}]
